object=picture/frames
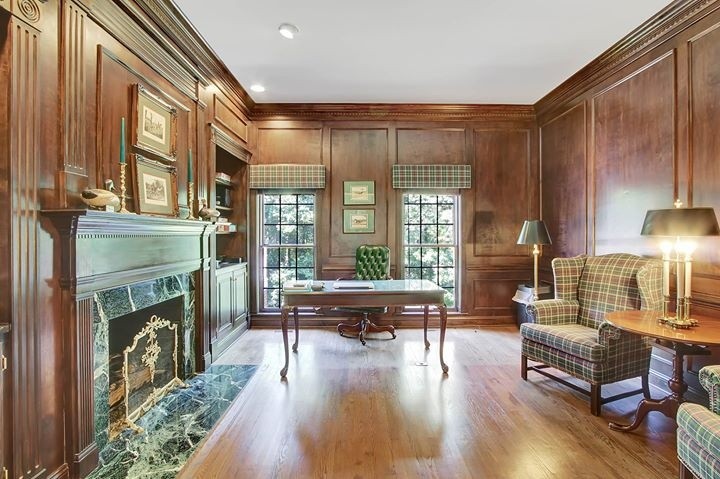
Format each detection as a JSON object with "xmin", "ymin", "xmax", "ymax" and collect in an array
[
  {"xmin": 343, "ymin": 180, "xmax": 376, "ymax": 206},
  {"xmin": 131, "ymin": 83, "xmax": 177, "ymax": 161},
  {"xmin": 129, "ymin": 153, "xmax": 180, "ymax": 217},
  {"xmin": 342, "ymin": 209, "xmax": 376, "ymax": 234}
]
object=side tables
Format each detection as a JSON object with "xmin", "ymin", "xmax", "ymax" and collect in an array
[{"xmin": 512, "ymin": 289, "xmax": 554, "ymax": 326}]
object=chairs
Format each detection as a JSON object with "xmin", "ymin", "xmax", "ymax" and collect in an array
[
  {"xmin": 519, "ymin": 253, "xmax": 664, "ymax": 414},
  {"xmin": 337, "ymin": 244, "xmax": 396, "ymax": 345},
  {"xmin": 675, "ymin": 364, "xmax": 720, "ymax": 479}
]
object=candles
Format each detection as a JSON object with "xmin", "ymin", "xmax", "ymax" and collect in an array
[
  {"xmin": 187, "ymin": 148, "xmax": 193, "ymax": 184},
  {"xmin": 120, "ymin": 114, "xmax": 126, "ymax": 163}
]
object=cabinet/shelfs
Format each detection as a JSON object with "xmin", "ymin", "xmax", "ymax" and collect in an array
[{"xmin": 216, "ymin": 146, "xmax": 247, "ymax": 340}]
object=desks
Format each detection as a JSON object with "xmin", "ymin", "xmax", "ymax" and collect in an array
[
  {"xmin": 277, "ymin": 279, "xmax": 449, "ymax": 378},
  {"xmin": 605, "ymin": 310, "xmax": 720, "ymax": 433}
]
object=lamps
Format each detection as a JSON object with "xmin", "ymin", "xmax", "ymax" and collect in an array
[
  {"xmin": 279, "ymin": 24, "xmax": 298, "ymax": 39},
  {"xmin": 517, "ymin": 220, "xmax": 552, "ymax": 298},
  {"xmin": 640, "ymin": 208, "xmax": 720, "ymax": 327}
]
